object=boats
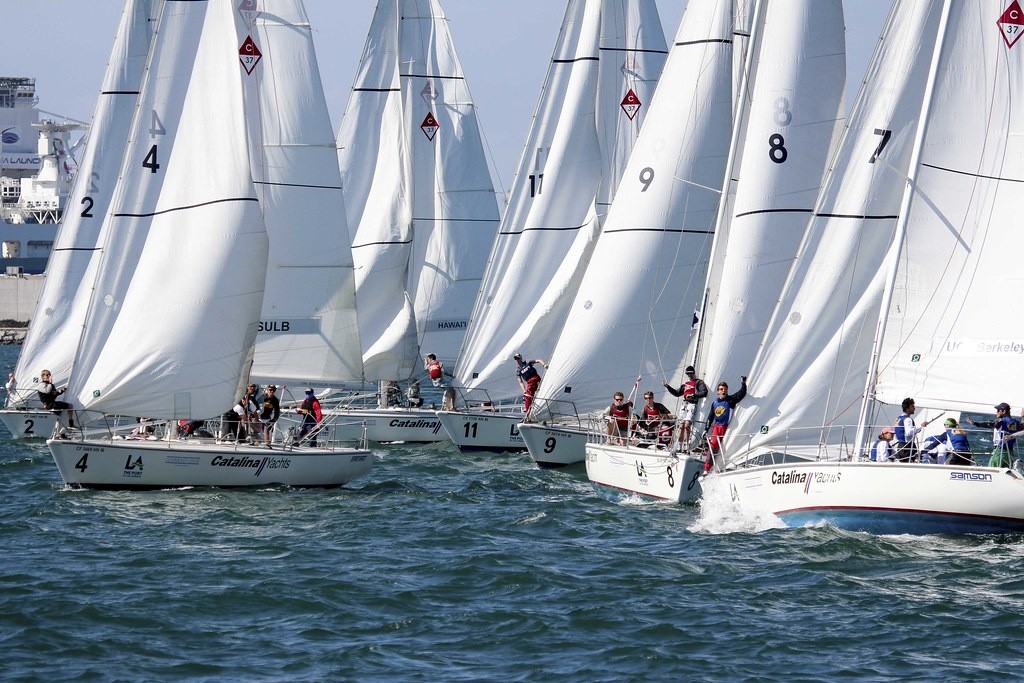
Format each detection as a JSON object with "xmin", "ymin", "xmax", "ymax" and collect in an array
[{"xmin": 1, "ymin": 76, "xmax": 92, "ymax": 327}]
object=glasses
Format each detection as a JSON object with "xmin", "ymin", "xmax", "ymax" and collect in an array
[
  {"xmin": 616, "ymin": 399, "xmax": 623, "ymax": 401},
  {"xmin": 719, "ymin": 388, "xmax": 727, "ymax": 391},
  {"xmin": 944, "ymin": 423, "xmax": 948, "ymax": 426},
  {"xmin": 686, "ymin": 373, "xmax": 693, "ymax": 375},
  {"xmin": 515, "ymin": 358, "xmax": 519, "ymax": 360},
  {"xmin": 998, "ymin": 409, "xmax": 1000, "ymax": 412},
  {"xmin": 645, "ymin": 397, "xmax": 652, "ymax": 399}
]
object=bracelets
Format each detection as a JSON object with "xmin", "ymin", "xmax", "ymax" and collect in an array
[{"xmin": 543, "ymin": 363, "xmax": 547, "ymax": 367}]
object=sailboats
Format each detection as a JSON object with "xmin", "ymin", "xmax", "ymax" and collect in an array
[{"xmin": 0, "ymin": 0, "xmax": 1024, "ymax": 538}]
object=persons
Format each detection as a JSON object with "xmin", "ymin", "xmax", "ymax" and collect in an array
[
  {"xmin": 894, "ymin": 398, "xmax": 928, "ymax": 462},
  {"xmin": 871, "ymin": 427, "xmax": 896, "ymax": 461},
  {"xmin": 407, "ymin": 378, "xmax": 424, "ymax": 408},
  {"xmin": 229, "ymin": 384, "xmax": 281, "ymax": 449},
  {"xmin": 513, "ymin": 354, "xmax": 548, "ymax": 415},
  {"xmin": 290, "ymin": 389, "xmax": 322, "ymax": 447},
  {"xmin": 387, "ymin": 381, "xmax": 402, "ymax": 407},
  {"xmin": 919, "ymin": 418, "xmax": 971, "ymax": 465},
  {"xmin": 662, "ymin": 366, "xmax": 708, "ymax": 453},
  {"xmin": 6, "ymin": 372, "xmax": 15, "ymax": 395},
  {"xmin": 37, "ymin": 370, "xmax": 80, "ymax": 438},
  {"xmin": 1004, "ymin": 430, "xmax": 1024, "ymax": 440},
  {"xmin": 598, "ymin": 392, "xmax": 634, "ymax": 445},
  {"xmin": 177, "ymin": 420, "xmax": 204, "ymax": 436},
  {"xmin": 633, "ymin": 391, "xmax": 673, "ymax": 447},
  {"xmin": 967, "ymin": 403, "xmax": 1024, "ymax": 467},
  {"xmin": 424, "ymin": 354, "xmax": 460, "ymax": 412},
  {"xmin": 702, "ymin": 376, "xmax": 747, "ymax": 476}
]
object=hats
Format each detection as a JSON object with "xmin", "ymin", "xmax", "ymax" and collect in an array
[
  {"xmin": 995, "ymin": 403, "xmax": 1010, "ymax": 411},
  {"xmin": 513, "ymin": 354, "xmax": 522, "ymax": 358},
  {"xmin": 267, "ymin": 384, "xmax": 276, "ymax": 389},
  {"xmin": 304, "ymin": 388, "xmax": 313, "ymax": 392},
  {"xmin": 882, "ymin": 427, "xmax": 895, "ymax": 434}
]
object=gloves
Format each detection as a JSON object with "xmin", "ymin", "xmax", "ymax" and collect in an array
[
  {"xmin": 701, "ymin": 430, "xmax": 707, "ymax": 439},
  {"xmin": 741, "ymin": 375, "xmax": 747, "ymax": 383}
]
